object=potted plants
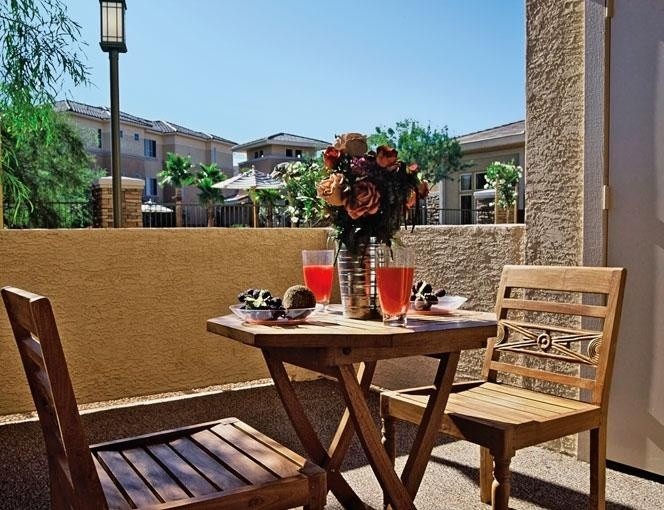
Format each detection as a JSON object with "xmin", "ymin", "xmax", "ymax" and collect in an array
[{"xmin": 484, "ymin": 157, "xmax": 523, "ymax": 223}]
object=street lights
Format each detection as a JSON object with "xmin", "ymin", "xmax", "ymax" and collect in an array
[{"xmin": 99, "ymin": 0, "xmax": 127, "ymax": 228}]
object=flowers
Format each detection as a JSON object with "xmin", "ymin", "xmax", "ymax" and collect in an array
[{"xmin": 274, "ymin": 132, "xmax": 430, "ymax": 309}]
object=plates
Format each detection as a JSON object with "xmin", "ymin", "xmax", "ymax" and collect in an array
[
  {"xmin": 228, "ymin": 304, "xmax": 323, "ymax": 325},
  {"xmin": 409, "ymin": 296, "xmax": 467, "ymax": 316}
]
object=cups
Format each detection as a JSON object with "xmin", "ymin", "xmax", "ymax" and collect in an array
[
  {"xmin": 375, "ymin": 248, "xmax": 414, "ymax": 327},
  {"xmin": 301, "ymin": 250, "xmax": 333, "ymax": 310}
]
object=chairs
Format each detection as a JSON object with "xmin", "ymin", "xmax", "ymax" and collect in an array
[
  {"xmin": 379, "ymin": 265, "xmax": 627, "ymax": 509},
  {"xmin": 0, "ymin": 285, "xmax": 328, "ymax": 510}
]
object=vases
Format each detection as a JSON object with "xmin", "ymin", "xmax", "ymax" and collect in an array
[{"xmin": 333, "ymin": 227, "xmax": 386, "ymax": 319}]
